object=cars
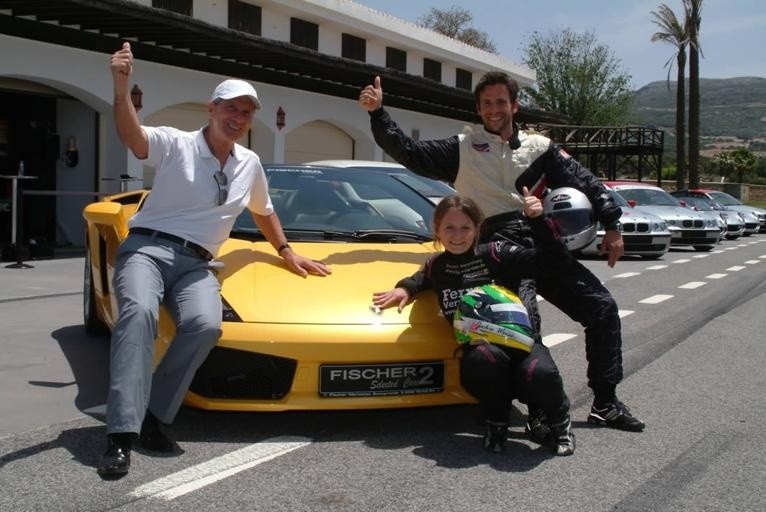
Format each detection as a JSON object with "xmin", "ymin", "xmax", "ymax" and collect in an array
[
  {"xmin": 581, "ymin": 184, "xmax": 671, "ymax": 258},
  {"xmin": 669, "ymin": 190, "xmax": 759, "ymax": 239},
  {"xmin": 605, "ymin": 186, "xmax": 722, "ymax": 253}
]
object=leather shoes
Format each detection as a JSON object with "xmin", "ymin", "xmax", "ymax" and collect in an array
[
  {"xmin": 97, "ymin": 437, "xmax": 132, "ymax": 479},
  {"xmin": 140, "ymin": 430, "xmax": 186, "ymax": 458}
]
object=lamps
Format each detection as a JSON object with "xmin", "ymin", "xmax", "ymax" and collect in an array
[
  {"xmin": 131, "ymin": 83, "xmax": 144, "ymax": 114},
  {"xmin": 276, "ymin": 106, "xmax": 286, "ymax": 132}
]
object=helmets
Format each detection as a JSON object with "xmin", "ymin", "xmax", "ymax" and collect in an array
[
  {"xmin": 453, "ymin": 283, "xmax": 536, "ymax": 355},
  {"xmin": 542, "ymin": 185, "xmax": 597, "ymax": 253}
]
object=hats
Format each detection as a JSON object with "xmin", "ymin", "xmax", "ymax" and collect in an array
[{"xmin": 209, "ymin": 77, "xmax": 263, "ymax": 111}]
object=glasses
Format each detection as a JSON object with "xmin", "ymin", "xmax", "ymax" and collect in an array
[{"xmin": 213, "ymin": 170, "xmax": 229, "ymax": 206}]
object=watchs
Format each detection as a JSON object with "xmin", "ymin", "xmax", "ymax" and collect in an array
[
  {"xmin": 603, "ymin": 222, "xmax": 625, "ymax": 232},
  {"xmin": 274, "ymin": 243, "xmax": 294, "ymax": 256}
]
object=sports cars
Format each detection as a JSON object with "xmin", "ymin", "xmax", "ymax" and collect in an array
[
  {"xmin": 83, "ymin": 160, "xmax": 520, "ymax": 413},
  {"xmin": 299, "ymin": 159, "xmax": 458, "ymax": 239}
]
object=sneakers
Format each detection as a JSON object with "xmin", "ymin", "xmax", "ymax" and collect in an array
[
  {"xmin": 586, "ymin": 398, "xmax": 644, "ymax": 433},
  {"xmin": 554, "ymin": 419, "xmax": 576, "ymax": 456},
  {"xmin": 524, "ymin": 410, "xmax": 555, "ymax": 445},
  {"xmin": 482, "ymin": 424, "xmax": 504, "ymax": 452}
]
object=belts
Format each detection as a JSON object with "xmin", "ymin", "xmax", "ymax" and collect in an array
[{"xmin": 126, "ymin": 225, "xmax": 216, "ymax": 268}]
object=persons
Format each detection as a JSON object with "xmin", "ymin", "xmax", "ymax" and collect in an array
[
  {"xmin": 357, "ymin": 69, "xmax": 648, "ymax": 433},
  {"xmin": 371, "ymin": 183, "xmax": 577, "ymax": 460},
  {"xmin": 97, "ymin": 37, "xmax": 332, "ymax": 478}
]
